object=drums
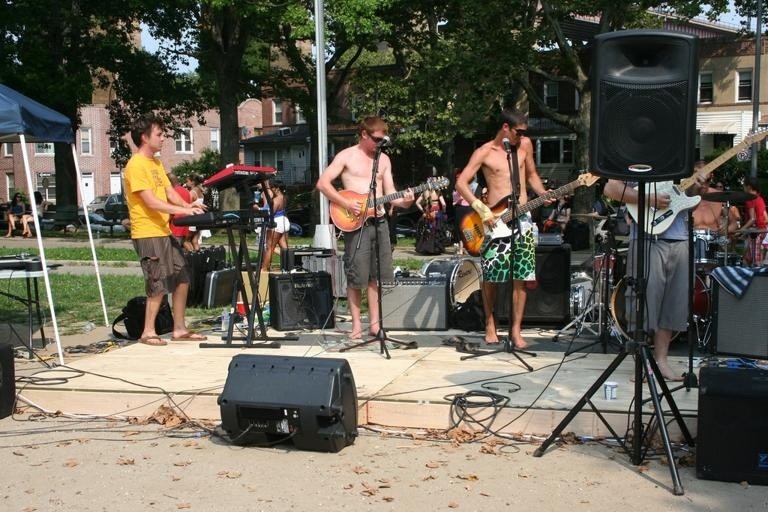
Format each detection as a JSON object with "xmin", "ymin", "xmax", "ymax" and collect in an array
[
  {"xmin": 610, "ymin": 270, "xmax": 711, "ymax": 350},
  {"xmin": 421, "ymin": 257, "xmax": 483, "ymax": 311},
  {"xmin": 693, "ymin": 226, "xmax": 719, "ymax": 265}
]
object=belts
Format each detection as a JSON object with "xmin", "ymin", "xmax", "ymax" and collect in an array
[{"xmin": 365, "ymin": 215, "xmax": 385, "ymax": 227}]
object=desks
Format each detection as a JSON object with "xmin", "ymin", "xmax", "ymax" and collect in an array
[{"xmin": 0, "ymin": 266, "xmax": 49, "ymax": 362}]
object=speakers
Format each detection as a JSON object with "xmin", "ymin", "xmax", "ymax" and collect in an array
[
  {"xmin": 591, "ymin": 30, "xmax": 700, "ymax": 183},
  {"xmin": 216, "ymin": 354, "xmax": 359, "ymax": 454},
  {"xmin": 696, "ymin": 366, "xmax": 768, "ymax": 486},
  {"xmin": 0, "ymin": 343, "xmax": 17, "ymax": 420},
  {"xmin": 269, "ymin": 270, "xmax": 334, "ymax": 331}
]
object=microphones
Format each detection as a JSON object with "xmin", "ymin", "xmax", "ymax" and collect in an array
[
  {"xmin": 377, "ymin": 135, "xmax": 391, "ymax": 150},
  {"xmin": 502, "ymin": 137, "xmax": 512, "ymax": 154}
]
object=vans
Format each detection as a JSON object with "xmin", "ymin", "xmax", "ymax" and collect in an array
[{"xmin": 86, "ymin": 192, "xmax": 128, "ymax": 221}]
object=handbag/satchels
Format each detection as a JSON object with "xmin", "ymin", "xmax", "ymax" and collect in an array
[
  {"xmin": 543, "ymin": 219, "xmax": 561, "ymax": 235},
  {"xmin": 111, "ymin": 294, "xmax": 174, "ymax": 341},
  {"xmin": 564, "ymin": 219, "xmax": 591, "ymax": 252}
]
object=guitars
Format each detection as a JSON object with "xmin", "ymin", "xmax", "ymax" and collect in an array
[
  {"xmin": 626, "ymin": 125, "xmax": 767, "ymax": 236},
  {"xmin": 330, "ymin": 176, "xmax": 451, "ymax": 233},
  {"xmin": 450, "ymin": 173, "xmax": 592, "ymax": 257}
]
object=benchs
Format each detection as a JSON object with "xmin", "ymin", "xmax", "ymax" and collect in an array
[
  {"xmin": 1, "ymin": 205, "xmax": 77, "ymax": 239},
  {"xmin": 100, "ymin": 204, "xmax": 129, "ymax": 237}
]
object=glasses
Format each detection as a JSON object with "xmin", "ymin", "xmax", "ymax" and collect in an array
[
  {"xmin": 366, "ymin": 130, "xmax": 383, "ymax": 142},
  {"xmin": 512, "ymin": 127, "xmax": 529, "ymax": 136},
  {"xmin": 16, "ymin": 195, "xmax": 22, "ymax": 198}
]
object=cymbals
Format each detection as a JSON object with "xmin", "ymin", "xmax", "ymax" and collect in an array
[
  {"xmin": 568, "ymin": 210, "xmax": 616, "ymax": 219},
  {"xmin": 736, "ymin": 227, "xmax": 768, "ymax": 233},
  {"xmin": 701, "ymin": 191, "xmax": 758, "ymax": 204}
]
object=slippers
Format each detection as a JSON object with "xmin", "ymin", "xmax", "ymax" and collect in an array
[
  {"xmin": 171, "ymin": 332, "xmax": 208, "ymax": 341},
  {"xmin": 138, "ymin": 337, "xmax": 167, "ymax": 345}
]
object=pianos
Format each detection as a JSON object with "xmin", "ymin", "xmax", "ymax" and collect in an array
[{"xmin": 174, "ymin": 208, "xmax": 271, "ymax": 226}]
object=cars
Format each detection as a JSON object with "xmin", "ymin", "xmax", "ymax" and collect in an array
[
  {"xmin": 278, "ymin": 204, "xmax": 417, "ymax": 238},
  {"xmin": 28, "ymin": 208, "xmax": 124, "ymax": 233},
  {"xmin": 592, "ymin": 212, "xmax": 618, "ymax": 245},
  {"xmin": 0, "ymin": 199, "xmax": 34, "ymax": 230}
]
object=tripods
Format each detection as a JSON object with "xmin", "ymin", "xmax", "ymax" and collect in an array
[
  {"xmin": 530, "ymin": 212, "xmax": 695, "ymax": 499},
  {"xmin": 458, "ymin": 212, "xmax": 540, "ymax": 373},
  {"xmin": 549, "ymin": 271, "xmax": 610, "ymax": 344},
  {"xmin": 565, "ymin": 252, "xmax": 624, "ymax": 357},
  {"xmin": 337, "ymin": 221, "xmax": 419, "ymax": 360}
]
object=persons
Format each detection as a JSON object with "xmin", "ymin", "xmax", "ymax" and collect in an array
[
  {"xmin": 316, "ymin": 117, "xmax": 414, "ymax": 338},
  {"xmin": 454, "ymin": 108, "xmax": 557, "ymax": 350},
  {"xmin": 602, "ymin": 170, "xmax": 713, "ymax": 382},
  {"xmin": 5, "ymin": 192, "xmax": 26, "ymax": 237},
  {"xmin": 122, "ymin": 116, "xmax": 207, "ymax": 344},
  {"xmin": 21, "ymin": 192, "xmax": 53, "ymax": 238}
]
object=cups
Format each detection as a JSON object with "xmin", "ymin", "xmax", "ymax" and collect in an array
[
  {"xmin": 604, "ymin": 381, "xmax": 618, "ymax": 399},
  {"xmin": 237, "ymin": 303, "xmax": 246, "ymax": 314},
  {"xmin": 254, "ymin": 192, "xmax": 261, "ymax": 203}
]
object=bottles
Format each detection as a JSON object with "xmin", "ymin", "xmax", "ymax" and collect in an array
[{"xmin": 222, "ymin": 308, "xmax": 229, "ymax": 331}]
